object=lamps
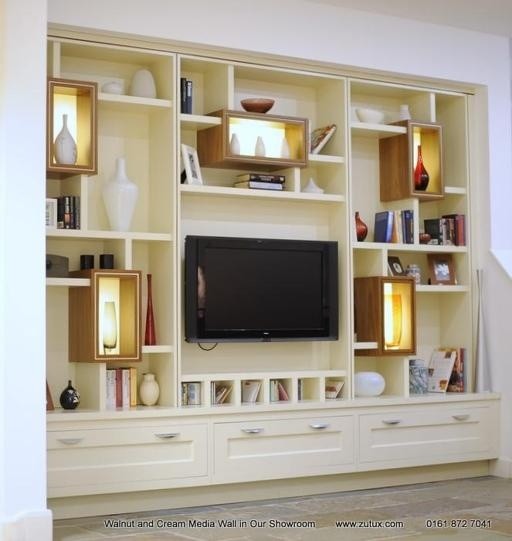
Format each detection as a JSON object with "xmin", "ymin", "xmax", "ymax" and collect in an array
[{"xmin": 102, "ymin": 300, "xmax": 119, "ymax": 354}]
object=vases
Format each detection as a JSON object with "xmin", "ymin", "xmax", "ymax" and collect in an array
[
  {"xmin": 135, "ymin": 368, "xmax": 162, "ymax": 410},
  {"xmin": 231, "ymin": 131, "xmax": 244, "ymax": 159},
  {"xmin": 412, "ymin": 142, "xmax": 432, "ymax": 195},
  {"xmin": 102, "ymin": 156, "xmax": 145, "ymax": 233},
  {"xmin": 253, "ymin": 134, "xmax": 269, "ymax": 159},
  {"xmin": 53, "ymin": 98, "xmax": 81, "ymax": 168},
  {"xmin": 355, "ymin": 369, "xmax": 385, "ymax": 399},
  {"xmin": 280, "ymin": 137, "xmax": 292, "ymax": 160}
]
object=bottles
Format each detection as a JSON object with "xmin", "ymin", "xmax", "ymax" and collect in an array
[
  {"xmin": 101, "ymin": 301, "xmax": 118, "ymax": 357},
  {"xmin": 471, "ymin": 268, "xmax": 492, "ymax": 393},
  {"xmin": 406, "ymin": 264, "xmax": 420, "ymax": 285},
  {"xmin": 400, "ymin": 104, "xmax": 411, "ymax": 120},
  {"xmin": 139, "ymin": 373, "xmax": 160, "ymax": 407},
  {"xmin": 59, "ymin": 381, "xmax": 81, "ymax": 411},
  {"xmin": 302, "ymin": 179, "xmax": 324, "ymax": 194},
  {"xmin": 412, "ymin": 144, "xmax": 429, "ymax": 192},
  {"xmin": 229, "ymin": 133, "xmax": 291, "ymax": 160},
  {"xmin": 54, "ymin": 69, "xmax": 157, "ymax": 235},
  {"xmin": 355, "ymin": 212, "xmax": 369, "ymax": 243},
  {"xmin": 144, "ymin": 273, "xmax": 156, "ymax": 346}
]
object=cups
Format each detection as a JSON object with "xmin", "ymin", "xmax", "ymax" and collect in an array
[{"xmin": 80, "ymin": 253, "xmax": 115, "ymax": 271}]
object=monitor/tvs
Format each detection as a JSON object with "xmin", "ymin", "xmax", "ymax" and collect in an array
[{"xmin": 184, "ymin": 235, "xmax": 339, "ymax": 343}]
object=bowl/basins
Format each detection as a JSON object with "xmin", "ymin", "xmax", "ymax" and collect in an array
[
  {"xmin": 419, "ymin": 233, "xmax": 431, "ymax": 244},
  {"xmin": 355, "ymin": 108, "xmax": 385, "ymax": 124},
  {"xmin": 239, "ymin": 97, "xmax": 275, "ymax": 113}
]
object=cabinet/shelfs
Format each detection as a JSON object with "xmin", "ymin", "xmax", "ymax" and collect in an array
[
  {"xmin": 50, "ymin": 36, "xmax": 178, "ymax": 416},
  {"xmin": 46, "ymin": 393, "xmax": 497, "ymax": 502},
  {"xmin": 346, "ymin": 75, "xmax": 482, "ymax": 403},
  {"xmin": 176, "ymin": 50, "xmax": 347, "ymax": 415}
]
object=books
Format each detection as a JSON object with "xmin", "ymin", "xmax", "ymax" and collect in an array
[
  {"xmin": 372, "ymin": 210, "xmax": 465, "ymax": 247},
  {"xmin": 425, "ymin": 352, "xmax": 456, "ymax": 393},
  {"xmin": 105, "ymin": 367, "xmax": 137, "ymax": 410},
  {"xmin": 232, "ymin": 181, "xmax": 283, "ymax": 192},
  {"xmin": 180, "ymin": 78, "xmax": 187, "ymax": 115},
  {"xmin": 186, "ymin": 81, "xmax": 193, "ymax": 116},
  {"xmin": 180, "ymin": 379, "xmax": 345, "ymax": 406},
  {"xmin": 233, "ymin": 173, "xmax": 285, "ymax": 184},
  {"xmin": 437, "ymin": 348, "xmax": 465, "ymax": 393},
  {"xmin": 45, "ymin": 195, "xmax": 80, "ymax": 231},
  {"xmin": 310, "ymin": 125, "xmax": 337, "ymax": 156}
]
